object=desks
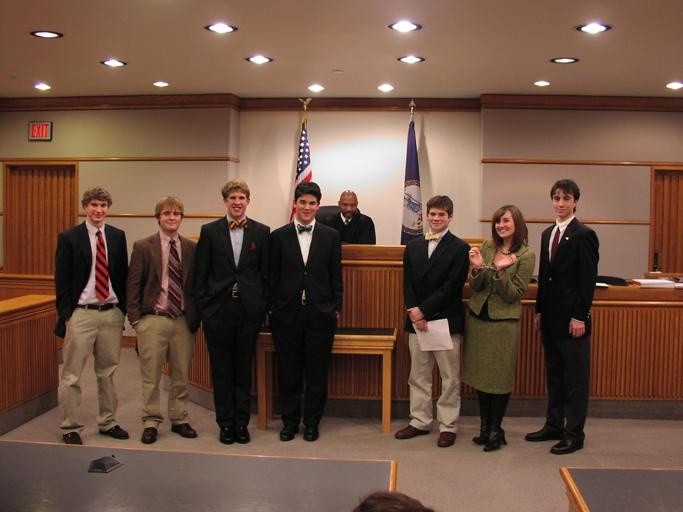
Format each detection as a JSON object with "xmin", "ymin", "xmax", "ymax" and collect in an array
[
  {"xmin": 0, "ymin": 293, "xmax": 61, "ymax": 436},
  {"xmin": 560, "ymin": 465, "xmax": 683, "ymax": 512},
  {"xmin": 0, "ymin": 438, "xmax": 395, "ymax": 512},
  {"xmin": 253, "ymin": 326, "xmax": 398, "ymax": 434}
]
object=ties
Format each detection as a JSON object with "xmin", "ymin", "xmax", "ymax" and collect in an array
[
  {"xmin": 95, "ymin": 230, "xmax": 110, "ymax": 302},
  {"xmin": 551, "ymin": 227, "xmax": 560, "ymax": 262},
  {"xmin": 168, "ymin": 239, "xmax": 182, "ymax": 320}
]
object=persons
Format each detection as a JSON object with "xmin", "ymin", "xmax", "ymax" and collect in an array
[
  {"xmin": 352, "ymin": 488, "xmax": 434, "ymax": 512},
  {"xmin": 126, "ymin": 196, "xmax": 200, "ymax": 446},
  {"xmin": 322, "ymin": 189, "xmax": 377, "ymax": 245},
  {"xmin": 394, "ymin": 194, "xmax": 472, "ymax": 448},
  {"xmin": 524, "ymin": 178, "xmax": 600, "ymax": 456},
  {"xmin": 182, "ymin": 176, "xmax": 271, "ymax": 446},
  {"xmin": 467, "ymin": 202, "xmax": 537, "ymax": 453},
  {"xmin": 265, "ymin": 182, "xmax": 346, "ymax": 445},
  {"xmin": 51, "ymin": 184, "xmax": 130, "ymax": 445}
]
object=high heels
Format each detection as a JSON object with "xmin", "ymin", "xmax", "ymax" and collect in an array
[
  {"xmin": 473, "ymin": 430, "xmax": 489, "ymax": 445},
  {"xmin": 484, "ymin": 428, "xmax": 506, "ymax": 451}
]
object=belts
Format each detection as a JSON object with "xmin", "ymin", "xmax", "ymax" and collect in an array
[
  {"xmin": 147, "ymin": 309, "xmax": 186, "ymax": 317},
  {"xmin": 75, "ymin": 302, "xmax": 119, "ymax": 311},
  {"xmin": 228, "ymin": 290, "xmax": 239, "ymax": 298}
]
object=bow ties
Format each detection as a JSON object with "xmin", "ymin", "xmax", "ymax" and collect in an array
[
  {"xmin": 298, "ymin": 224, "xmax": 313, "ymax": 233},
  {"xmin": 425, "ymin": 232, "xmax": 441, "ymax": 240},
  {"xmin": 228, "ymin": 219, "xmax": 248, "ymax": 230}
]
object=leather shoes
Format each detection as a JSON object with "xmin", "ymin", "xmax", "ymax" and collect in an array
[
  {"xmin": 396, "ymin": 425, "xmax": 429, "ymax": 439},
  {"xmin": 526, "ymin": 428, "xmax": 564, "ymax": 441},
  {"xmin": 551, "ymin": 439, "xmax": 583, "ymax": 453},
  {"xmin": 63, "ymin": 422, "xmax": 319, "ymax": 443},
  {"xmin": 438, "ymin": 431, "xmax": 456, "ymax": 447}
]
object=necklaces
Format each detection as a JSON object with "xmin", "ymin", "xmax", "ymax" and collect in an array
[{"xmin": 499, "ymin": 242, "xmax": 514, "ymax": 255}]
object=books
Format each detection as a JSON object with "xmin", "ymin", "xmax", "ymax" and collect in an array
[{"xmin": 630, "ymin": 277, "xmax": 675, "ymax": 289}]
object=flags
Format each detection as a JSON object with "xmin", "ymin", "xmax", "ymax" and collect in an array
[
  {"xmin": 399, "ymin": 120, "xmax": 425, "ymax": 246},
  {"xmin": 290, "ymin": 115, "xmax": 313, "ymax": 222}
]
